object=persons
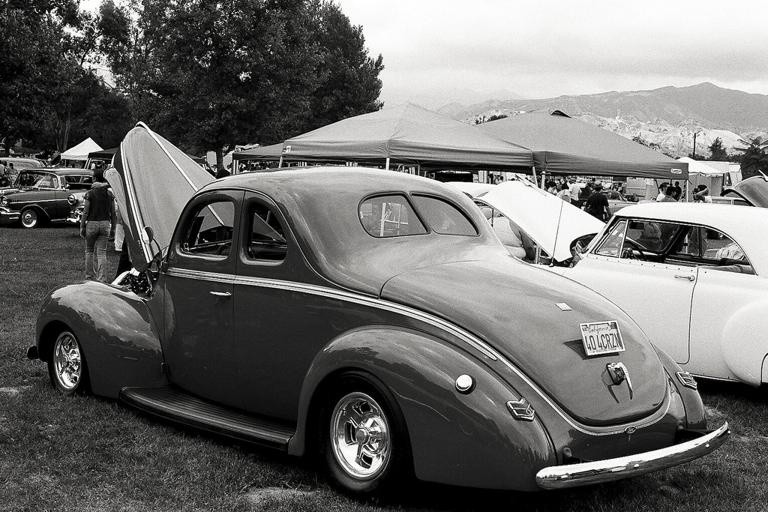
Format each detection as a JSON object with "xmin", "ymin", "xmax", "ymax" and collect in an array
[
  {"xmin": 691, "ymin": 187, "xmax": 699, "ymax": 203},
  {"xmin": 79, "ymin": 167, "xmax": 116, "ymax": 285},
  {"xmin": 5, "ymin": 161, "xmax": 19, "ymax": 188},
  {"xmin": 671, "ymin": 181, "xmax": 682, "ymax": 201},
  {"xmin": 657, "ymin": 184, "xmax": 661, "ymax": 194},
  {"xmin": 695, "ymin": 184, "xmax": 711, "ymax": 203},
  {"xmin": 0, "ymin": 159, "xmax": 5, "ymax": 178},
  {"xmin": 666, "ymin": 183, "xmax": 675, "ymax": 196},
  {"xmin": 486, "ymin": 209, "xmax": 535, "ymax": 263},
  {"xmin": 492, "ymin": 172, "xmax": 638, "ymax": 220},
  {"xmin": 656, "ymin": 182, "xmax": 677, "ymax": 202}
]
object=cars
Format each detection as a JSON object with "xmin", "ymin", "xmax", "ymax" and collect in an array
[
  {"xmin": 0, "ymin": 148, "xmax": 117, "ymax": 229},
  {"xmin": 472, "ymin": 177, "xmax": 767, "ymax": 385},
  {"xmin": 27, "ymin": 119, "xmax": 730, "ymax": 493}
]
